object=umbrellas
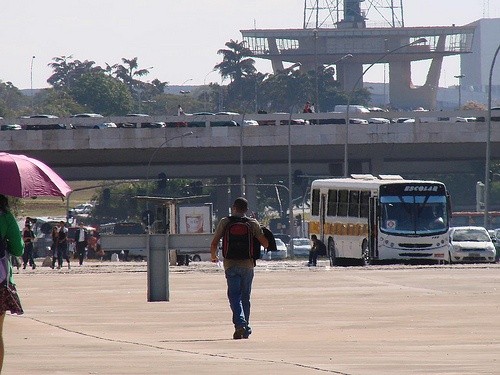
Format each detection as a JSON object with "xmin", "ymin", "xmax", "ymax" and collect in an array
[{"xmin": 1, "ymin": 152, "xmax": 72, "ymax": 200}]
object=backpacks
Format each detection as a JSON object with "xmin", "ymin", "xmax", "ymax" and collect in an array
[{"xmin": 221, "ymin": 216, "xmax": 257, "ymax": 260}]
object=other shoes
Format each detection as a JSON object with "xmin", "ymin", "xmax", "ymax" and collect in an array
[{"xmin": 233, "ymin": 323, "xmax": 251, "ymax": 339}]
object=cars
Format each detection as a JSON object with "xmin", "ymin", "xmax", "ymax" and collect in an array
[
  {"xmin": 260, "ymin": 233, "xmax": 290, "ymax": 260},
  {"xmin": 189, "ymin": 238, "xmax": 224, "ymax": 261},
  {"xmin": 0, "ymin": 106, "xmax": 500, "ymax": 132},
  {"xmin": 448, "ymin": 226, "xmax": 497, "ymax": 265},
  {"xmin": 287, "ymin": 238, "xmax": 313, "ymax": 259},
  {"xmin": 22, "ymin": 203, "xmax": 147, "ymax": 260}
]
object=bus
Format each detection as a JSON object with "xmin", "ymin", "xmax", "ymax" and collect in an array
[
  {"xmin": 308, "ymin": 173, "xmax": 452, "ymax": 267},
  {"xmin": 449, "ymin": 212, "xmax": 500, "ymax": 229}
]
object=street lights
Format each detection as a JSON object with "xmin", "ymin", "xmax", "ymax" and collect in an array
[
  {"xmin": 290, "ymin": 54, "xmax": 353, "ymax": 238},
  {"xmin": 238, "ymin": 62, "xmax": 302, "ymax": 197},
  {"xmin": 29, "ymin": 56, "xmax": 36, "ymax": 116},
  {"xmin": 344, "ymin": 38, "xmax": 428, "ymax": 177}
]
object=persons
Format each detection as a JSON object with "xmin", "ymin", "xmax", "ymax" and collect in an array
[
  {"xmin": 74, "ymin": 222, "xmax": 88, "ymax": 266},
  {"xmin": 303, "ymin": 101, "xmax": 318, "ymax": 125},
  {"xmin": 22, "ymin": 224, "xmax": 36, "ymax": 270},
  {"xmin": 50, "ymin": 226, "xmax": 63, "ymax": 269},
  {"xmin": 209, "ymin": 197, "xmax": 268, "ymax": 339},
  {"xmin": 1, "ymin": 194, "xmax": 25, "ymax": 373},
  {"xmin": 56, "ymin": 221, "xmax": 71, "ymax": 269},
  {"xmin": 176, "ymin": 104, "xmax": 187, "ymax": 127},
  {"xmin": 22, "ymin": 221, "xmax": 33, "ymax": 266},
  {"xmin": 305, "ymin": 235, "xmax": 326, "ymax": 266}
]
